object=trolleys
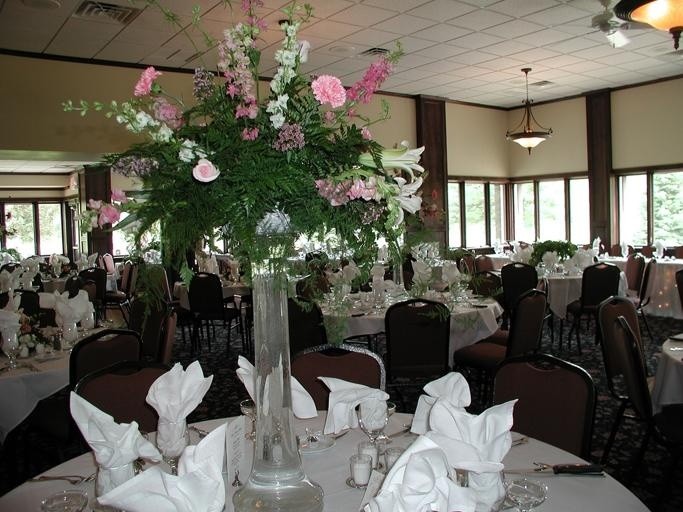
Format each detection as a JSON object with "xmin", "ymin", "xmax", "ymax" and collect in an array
[{"xmin": 582, "ymin": 0, "xmax": 657, "ymax": 50}]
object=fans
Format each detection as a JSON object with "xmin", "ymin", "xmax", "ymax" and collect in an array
[{"xmin": 582, "ymin": 0, "xmax": 657, "ymax": 50}]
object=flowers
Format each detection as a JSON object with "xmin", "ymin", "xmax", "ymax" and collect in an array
[{"xmin": 57, "ymin": 0, "xmax": 447, "ymax": 349}]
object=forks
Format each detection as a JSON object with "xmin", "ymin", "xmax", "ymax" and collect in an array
[{"xmin": 27, "ymin": 475, "xmax": 87, "ymax": 486}]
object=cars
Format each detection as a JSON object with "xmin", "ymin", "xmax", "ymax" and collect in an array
[
  {"xmin": 36, "ymin": 416, "xmax": 192, "ymax": 511},
  {"xmin": 503, "ymin": 477, "xmax": 548, "ymax": 511},
  {"xmin": 348, "ymin": 400, "xmax": 406, "ymax": 489}
]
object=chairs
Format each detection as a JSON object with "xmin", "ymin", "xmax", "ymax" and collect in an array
[{"xmin": 0, "ymin": 238, "xmax": 683, "ymax": 512}]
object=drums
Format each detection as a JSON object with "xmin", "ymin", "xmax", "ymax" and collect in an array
[{"xmin": 502, "ymin": 463, "xmax": 605, "ymax": 476}]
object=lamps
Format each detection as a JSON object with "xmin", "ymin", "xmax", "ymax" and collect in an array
[
  {"xmin": 503, "ymin": 67, "xmax": 554, "ymax": 157},
  {"xmin": 613, "ymin": 1, "xmax": 682, "ymax": 52}
]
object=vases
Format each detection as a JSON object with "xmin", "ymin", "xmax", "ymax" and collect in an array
[{"xmin": 57, "ymin": 0, "xmax": 447, "ymax": 349}]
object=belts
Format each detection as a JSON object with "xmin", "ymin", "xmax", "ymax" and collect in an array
[
  {"xmin": 419, "ymin": 242, "xmax": 439, "ymax": 262},
  {"xmin": 653, "ymin": 252, "xmax": 663, "ymax": 262},
  {"xmin": 1, "ymin": 307, "xmax": 111, "ymax": 372},
  {"xmin": 622, "ymin": 251, "xmax": 627, "ymax": 260},
  {"xmin": 533, "ymin": 260, "xmax": 585, "ymax": 276},
  {"xmin": 324, "ymin": 281, "xmax": 475, "ymax": 315},
  {"xmin": 240, "ymin": 398, "xmax": 255, "ymax": 442},
  {"xmin": 54, "ymin": 270, "xmax": 60, "ymax": 281}
]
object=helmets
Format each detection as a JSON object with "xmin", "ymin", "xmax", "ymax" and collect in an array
[{"xmin": 296, "ymin": 433, "xmax": 337, "ymax": 454}]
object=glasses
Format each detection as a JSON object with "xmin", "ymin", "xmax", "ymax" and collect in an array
[
  {"xmin": 503, "ymin": 67, "xmax": 554, "ymax": 157},
  {"xmin": 613, "ymin": 1, "xmax": 682, "ymax": 52}
]
